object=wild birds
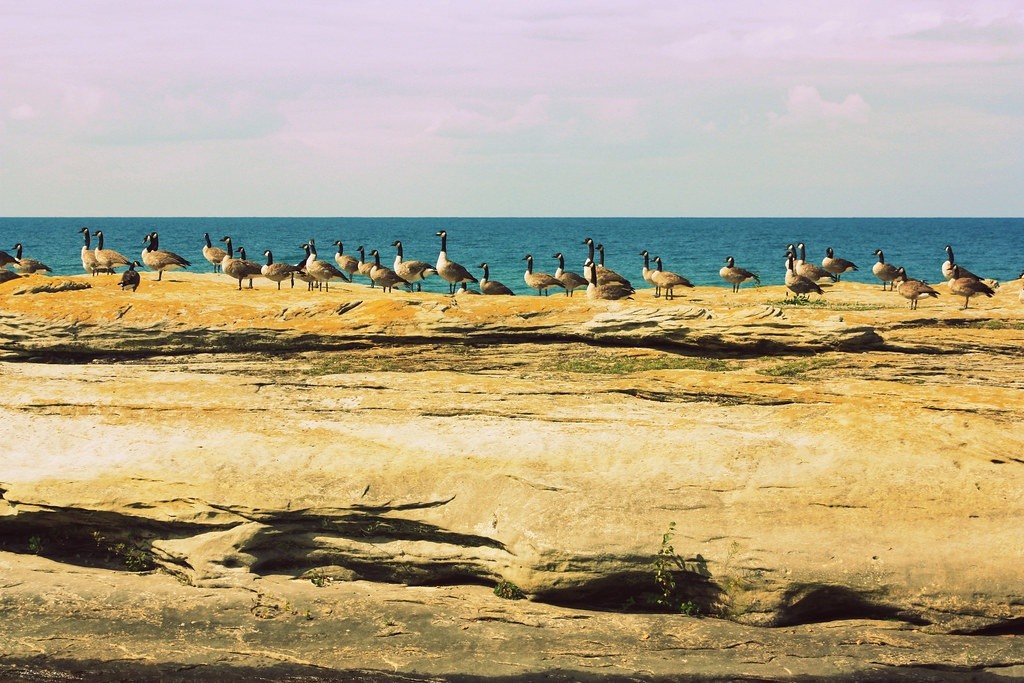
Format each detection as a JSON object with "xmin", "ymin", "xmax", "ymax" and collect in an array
[
  {"xmin": 941, "ymin": 245, "xmax": 985, "ymax": 281},
  {"xmin": 552, "ymin": 253, "xmax": 589, "ymax": 297},
  {"xmin": 0, "ymin": 227, "xmax": 412, "ymax": 294},
  {"xmin": 719, "ymin": 257, "xmax": 759, "ymax": 294},
  {"xmin": 435, "ymin": 230, "xmax": 478, "ymax": 294},
  {"xmin": 650, "ymin": 255, "xmax": 696, "ymax": 301},
  {"xmin": 1017, "ymin": 272, "xmax": 1024, "ymax": 304},
  {"xmin": 581, "ymin": 237, "xmax": 636, "ymax": 301},
  {"xmin": 894, "ymin": 267, "xmax": 941, "ymax": 310},
  {"xmin": 638, "ymin": 250, "xmax": 661, "ymax": 298},
  {"xmin": 522, "ymin": 255, "xmax": 567, "ymax": 296},
  {"xmin": 782, "ymin": 251, "xmax": 825, "ymax": 297},
  {"xmin": 822, "ymin": 247, "xmax": 859, "ymax": 282},
  {"xmin": 871, "ymin": 250, "xmax": 900, "ymax": 292},
  {"xmin": 947, "ymin": 265, "xmax": 996, "ymax": 309},
  {"xmin": 476, "ymin": 263, "xmax": 517, "ymax": 297},
  {"xmin": 456, "ymin": 281, "xmax": 481, "ymax": 295},
  {"xmin": 795, "ymin": 243, "xmax": 837, "ymax": 283},
  {"xmin": 784, "ymin": 244, "xmax": 799, "ymax": 269},
  {"xmin": 392, "ymin": 241, "xmax": 438, "ymax": 292}
]
